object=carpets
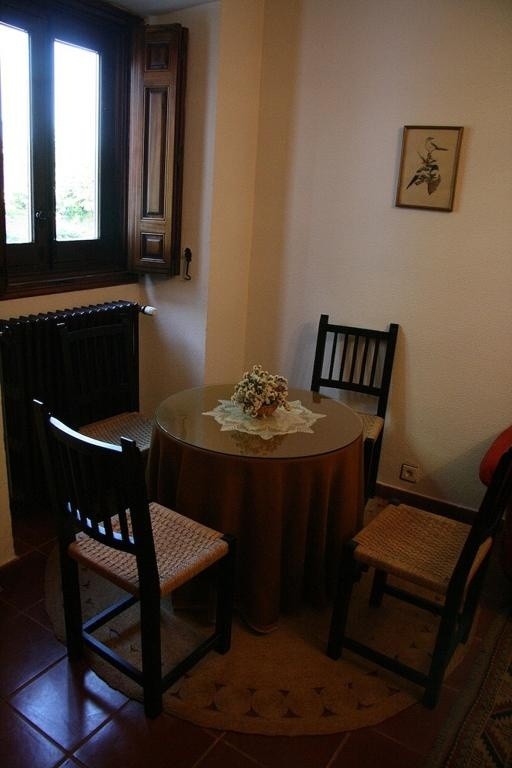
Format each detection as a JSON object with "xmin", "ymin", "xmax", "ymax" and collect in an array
[{"xmin": 45, "ymin": 498, "xmax": 484, "ymax": 736}]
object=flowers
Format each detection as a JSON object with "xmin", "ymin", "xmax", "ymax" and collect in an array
[{"xmin": 231, "ymin": 365, "xmax": 291, "ymax": 418}]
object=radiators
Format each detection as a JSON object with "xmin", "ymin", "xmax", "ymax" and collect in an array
[{"xmin": 1, "ymin": 300, "xmax": 153, "ymax": 534}]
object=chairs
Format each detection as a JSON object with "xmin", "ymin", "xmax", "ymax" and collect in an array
[
  {"xmin": 52, "ymin": 314, "xmax": 155, "ymax": 504},
  {"xmin": 31, "ymin": 399, "xmax": 233, "ymax": 720},
  {"xmin": 326, "ymin": 446, "xmax": 512, "ymax": 712},
  {"xmin": 310, "ymin": 313, "xmax": 401, "ymax": 505}
]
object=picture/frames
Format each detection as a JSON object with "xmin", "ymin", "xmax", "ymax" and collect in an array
[{"xmin": 394, "ymin": 125, "xmax": 464, "ymax": 213}]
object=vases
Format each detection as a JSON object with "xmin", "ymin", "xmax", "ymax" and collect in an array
[{"xmin": 256, "ymin": 400, "xmax": 277, "ymax": 418}]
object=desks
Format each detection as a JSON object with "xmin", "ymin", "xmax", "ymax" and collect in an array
[{"xmin": 156, "ymin": 383, "xmax": 364, "ymax": 635}]
